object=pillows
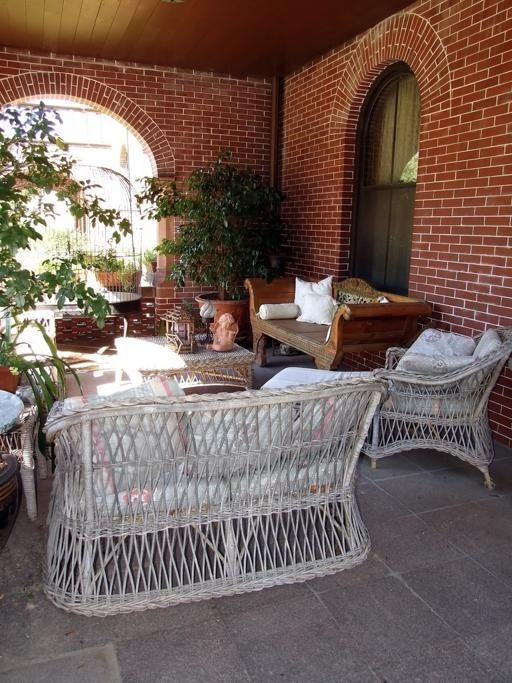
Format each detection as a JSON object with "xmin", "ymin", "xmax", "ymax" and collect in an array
[
  {"xmin": 293, "ymin": 283, "xmax": 340, "ymax": 325},
  {"xmin": 395, "ymin": 355, "xmax": 477, "ymax": 393},
  {"xmin": 258, "ymin": 300, "xmax": 300, "ymax": 320},
  {"xmin": 401, "ymin": 326, "xmax": 475, "ymax": 358},
  {"xmin": 258, "ymin": 376, "xmax": 371, "ymax": 455},
  {"xmin": 51, "ymin": 373, "xmax": 190, "ymax": 497},
  {"xmin": 187, "ymin": 391, "xmax": 299, "ymax": 476},
  {"xmin": 459, "ymin": 327, "xmax": 501, "ymax": 397},
  {"xmin": 293, "ymin": 274, "xmax": 334, "ymax": 315}
]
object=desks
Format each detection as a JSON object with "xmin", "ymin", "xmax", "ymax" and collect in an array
[{"xmin": 112, "ymin": 330, "xmax": 258, "ymax": 394}]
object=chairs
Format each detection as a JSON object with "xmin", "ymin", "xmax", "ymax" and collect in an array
[{"xmin": 258, "ymin": 321, "xmax": 512, "ymax": 491}]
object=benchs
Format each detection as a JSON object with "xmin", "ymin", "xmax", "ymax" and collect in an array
[
  {"xmin": 243, "ymin": 274, "xmax": 432, "ymax": 372},
  {"xmin": 0, "ymin": 382, "xmax": 49, "ymax": 522},
  {"xmin": 37, "ymin": 364, "xmax": 392, "ymax": 618}
]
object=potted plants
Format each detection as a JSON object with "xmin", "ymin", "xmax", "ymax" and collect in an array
[
  {"xmin": 95, "ymin": 258, "xmax": 143, "ymax": 292},
  {"xmin": 144, "ymin": 249, "xmax": 157, "ymax": 272},
  {"xmin": 132, "ymin": 149, "xmax": 299, "ymax": 337}
]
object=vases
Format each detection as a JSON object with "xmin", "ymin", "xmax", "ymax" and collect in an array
[{"xmin": 0, "ymin": 365, "xmax": 18, "ymax": 395}]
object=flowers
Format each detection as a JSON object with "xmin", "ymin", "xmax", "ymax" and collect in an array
[{"xmin": 0, "ymin": 344, "xmax": 29, "ymax": 375}]
